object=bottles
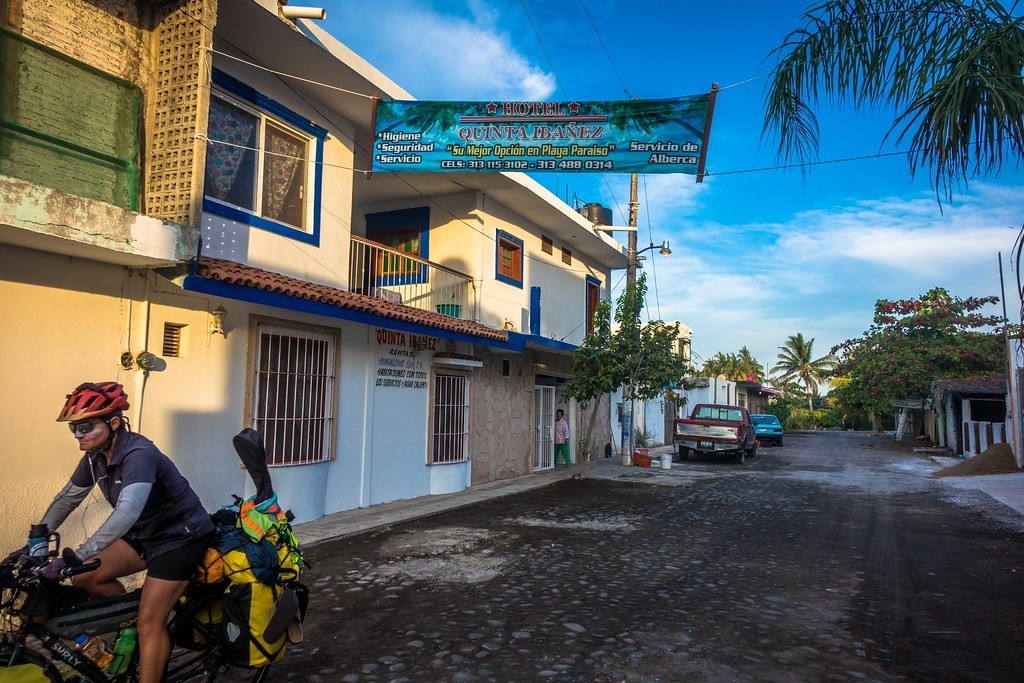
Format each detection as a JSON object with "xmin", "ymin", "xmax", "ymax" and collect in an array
[
  {"xmin": 75, "ymin": 634, "xmax": 114, "ymax": 669},
  {"xmin": 108, "ymin": 628, "xmax": 137, "ymax": 675}
]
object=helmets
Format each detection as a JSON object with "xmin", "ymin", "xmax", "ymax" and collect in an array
[{"xmin": 57, "ymin": 382, "xmax": 129, "ymax": 421}]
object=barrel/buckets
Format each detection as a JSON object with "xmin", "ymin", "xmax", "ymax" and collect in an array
[
  {"xmin": 660, "ymin": 454, "xmax": 672, "ymax": 470},
  {"xmin": 436, "ymin": 304, "xmax": 462, "ymax": 319}
]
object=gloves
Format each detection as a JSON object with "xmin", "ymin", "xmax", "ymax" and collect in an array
[
  {"xmin": 1, "ymin": 545, "xmax": 30, "ymax": 565},
  {"xmin": 38, "ymin": 558, "xmax": 68, "ymax": 588}
]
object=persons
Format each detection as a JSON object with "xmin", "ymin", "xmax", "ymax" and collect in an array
[
  {"xmin": 1, "ymin": 388, "xmax": 213, "ymax": 682},
  {"xmin": 549, "ymin": 409, "xmax": 572, "ymax": 470}
]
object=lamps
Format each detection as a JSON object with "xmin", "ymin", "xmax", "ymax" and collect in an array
[{"xmin": 638, "ymin": 239, "xmax": 671, "ymax": 254}]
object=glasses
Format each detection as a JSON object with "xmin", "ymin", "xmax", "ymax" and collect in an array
[{"xmin": 68, "ymin": 418, "xmax": 105, "ymax": 434}]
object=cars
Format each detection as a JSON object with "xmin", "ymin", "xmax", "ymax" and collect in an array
[{"xmin": 746, "ymin": 413, "xmax": 783, "ymax": 447}]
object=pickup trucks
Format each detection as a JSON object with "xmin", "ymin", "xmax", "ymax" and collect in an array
[{"xmin": 673, "ymin": 403, "xmax": 759, "ymax": 463}]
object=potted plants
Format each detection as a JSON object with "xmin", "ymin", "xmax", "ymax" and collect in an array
[{"xmin": 631, "ymin": 428, "xmax": 651, "ymax": 449}]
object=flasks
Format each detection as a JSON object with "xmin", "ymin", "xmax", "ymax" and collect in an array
[{"xmin": 29, "ymin": 524, "xmax": 60, "ymax": 564}]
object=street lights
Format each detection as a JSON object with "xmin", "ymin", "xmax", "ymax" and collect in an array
[{"xmin": 621, "ymin": 239, "xmax": 673, "ymax": 466}]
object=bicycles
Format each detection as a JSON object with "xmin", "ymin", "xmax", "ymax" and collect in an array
[{"xmin": 0, "ymin": 533, "xmax": 272, "ymax": 683}]
